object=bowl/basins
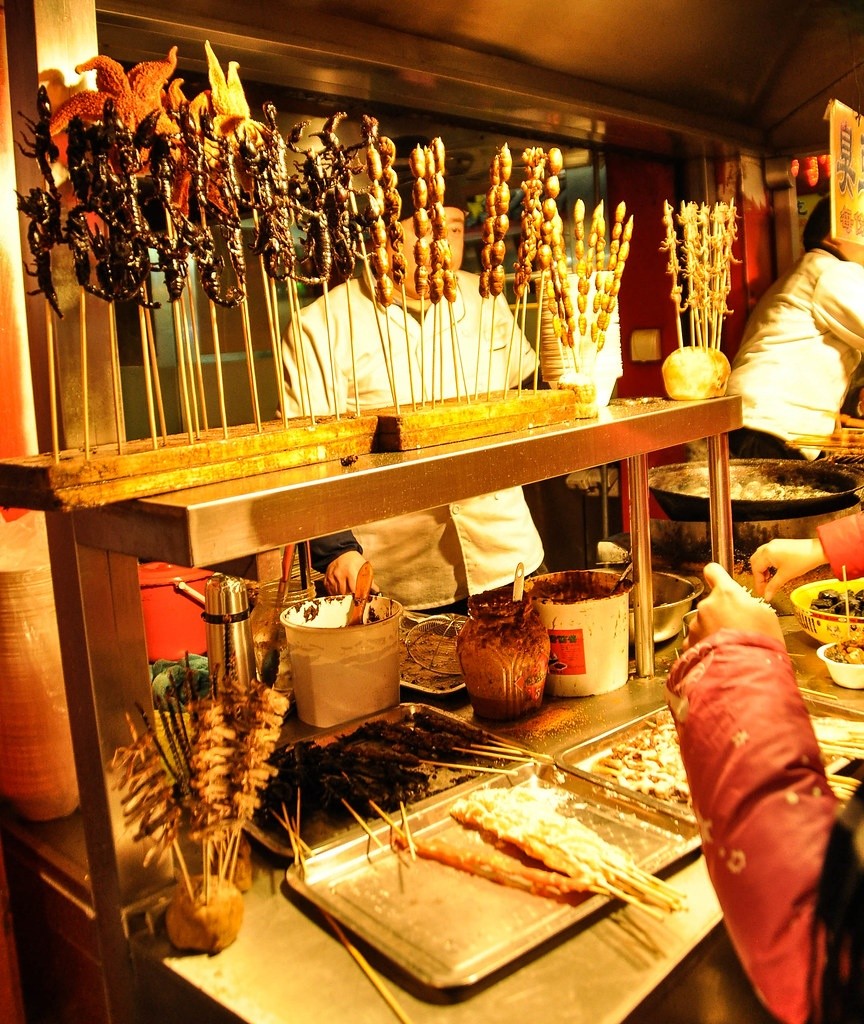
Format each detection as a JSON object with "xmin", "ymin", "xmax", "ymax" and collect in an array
[
  {"xmin": 614, "ymin": 572, "xmax": 704, "ymax": 645},
  {"xmin": 681, "ymin": 609, "xmax": 698, "ymax": 638},
  {"xmin": 790, "ymin": 577, "xmax": 864, "ymax": 646},
  {"xmin": 535, "ymin": 271, "xmax": 622, "ymax": 407},
  {"xmin": 816, "ymin": 643, "xmax": 864, "ymax": 689},
  {"xmin": 0, "ymin": 564, "xmax": 79, "ymax": 821}
]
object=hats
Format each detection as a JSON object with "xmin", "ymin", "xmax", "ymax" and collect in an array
[{"xmin": 364, "ymin": 131, "xmax": 465, "ymax": 226}]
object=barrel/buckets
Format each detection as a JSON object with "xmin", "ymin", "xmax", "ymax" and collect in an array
[
  {"xmin": 279, "ymin": 594, "xmax": 404, "ymax": 728},
  {"xmin": 521, "ymin": 570, "xmax": 634, "ymax": 696}
]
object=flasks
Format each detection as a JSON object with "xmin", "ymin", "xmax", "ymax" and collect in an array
[{"xmin": 201, "ymin": 573, "xmax": 256, "ymax": 702}]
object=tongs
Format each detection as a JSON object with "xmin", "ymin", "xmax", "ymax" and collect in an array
[{"xmin": 261, "ymin": 540, "xmax": 312, "ymax": 690}]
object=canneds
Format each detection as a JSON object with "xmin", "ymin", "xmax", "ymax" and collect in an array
[{"xmin": 456, "ymin": 587, "xmax": 551, "ymax": 722}]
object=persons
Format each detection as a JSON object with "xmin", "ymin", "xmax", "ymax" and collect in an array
[
  {"xmin": 276, "ymin": 132, "xmax": 549, "ymax": 617},
  {"xmin": 664, "ymin": 509, "xmax": 864, "ymax": 1024},
  {"xmin": 726, "ymin": 196, "xmax": 864, "ymax": 462}
]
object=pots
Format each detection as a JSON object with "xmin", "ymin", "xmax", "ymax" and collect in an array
[{"xmin": 648, "ymin": 459, "xmax": 864, "ymax": 523}]
